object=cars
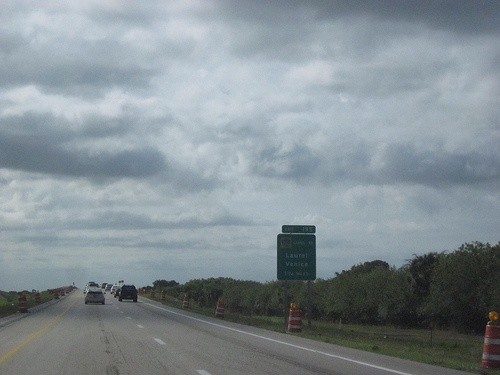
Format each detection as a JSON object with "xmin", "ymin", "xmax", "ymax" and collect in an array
[{"xmin": 83, "ymin": 281, "xmax": 138, "ymax": 305}]
